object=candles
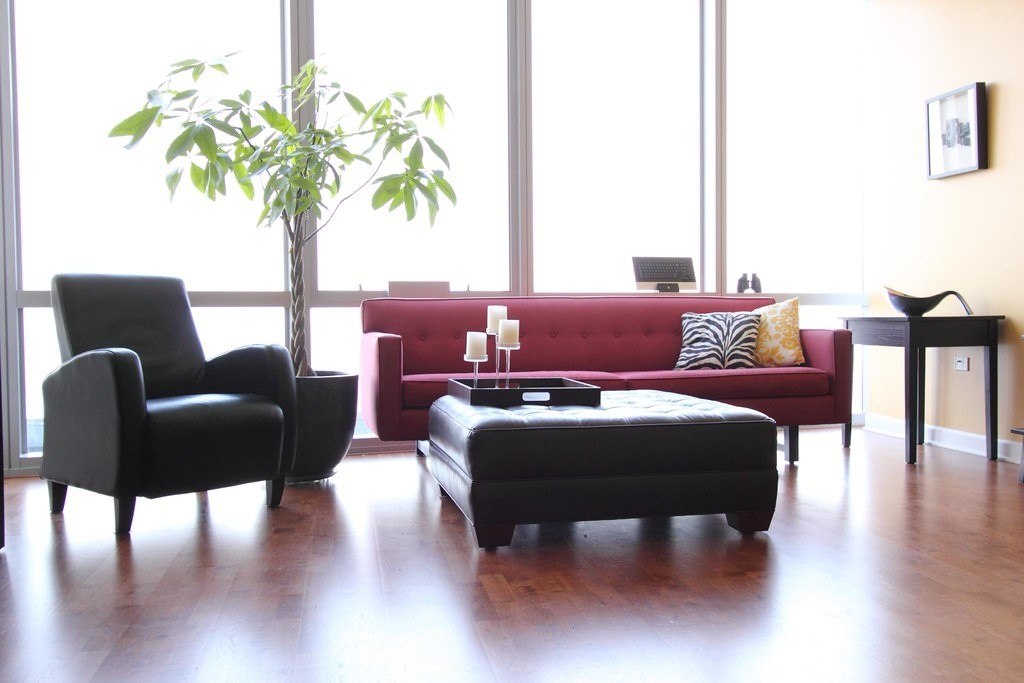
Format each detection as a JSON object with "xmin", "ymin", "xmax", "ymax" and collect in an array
[
  {"xmin": 487, "ymin": 304, "xmax": 508, "ymax": 329},
  {"xmin": 497, "ymin": 320, "xmax": 520, "ymax": 345},
  {"xmin": 466, "ymin": 331, "xmax": 487, "ymax": 354}
]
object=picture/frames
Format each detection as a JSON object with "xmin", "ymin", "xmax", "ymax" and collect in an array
[{"xmin": 925, "ymin": 80, "xmax": 989, "ymax": 179}]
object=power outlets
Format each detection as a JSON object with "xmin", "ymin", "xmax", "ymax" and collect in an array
[{"xmin": 953, "ymin": 356, "xmax": 971, "ymax": 371}]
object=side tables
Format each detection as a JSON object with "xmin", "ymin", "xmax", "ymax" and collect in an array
[{"xmin": 837, "ymin": 312, "xmax": 1007, "ymax": 464}]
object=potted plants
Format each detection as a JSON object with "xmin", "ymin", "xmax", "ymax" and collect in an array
[{"xmin": 109, "ymin": 50, "xmax": 456, "ymax": 485}]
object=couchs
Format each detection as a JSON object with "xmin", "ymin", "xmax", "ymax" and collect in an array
[
  {"xmin": 358, "ymin": 294, "xmax": 851, "ymax": 463},
  {"xmin": 40, "ymin": 270, "xmax": 297, "ymax": 536}
]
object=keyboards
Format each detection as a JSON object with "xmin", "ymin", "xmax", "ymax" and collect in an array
[{"xmin": 632, "ymin": 257, "xmax": 697, "ymax": 290}]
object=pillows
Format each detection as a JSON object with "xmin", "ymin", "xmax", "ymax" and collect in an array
[
  {"xmin": 676, "ymin": 310, "xmax": 763, "ymax": 369},
  {"xmin": 752, "ymin": 297, "xmax": 806, "ymax": 367}
]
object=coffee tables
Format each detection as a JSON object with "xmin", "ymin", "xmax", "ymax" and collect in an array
[{"xmin": 426, "ymin": 389, "xmax": 779, "ymax": 548}]
